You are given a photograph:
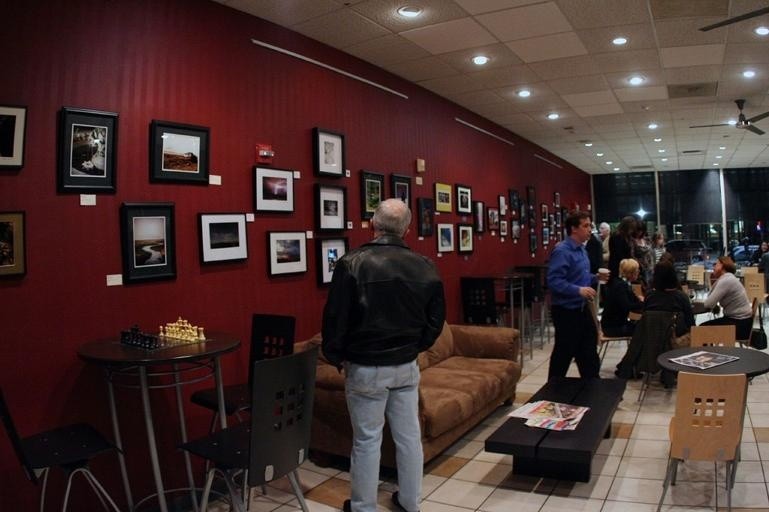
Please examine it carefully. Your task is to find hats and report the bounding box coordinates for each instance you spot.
[654,264,681,289]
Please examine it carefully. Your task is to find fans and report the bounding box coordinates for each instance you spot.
[696,5,767,30]
[688,99,769,139]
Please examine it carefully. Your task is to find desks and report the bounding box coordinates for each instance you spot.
[70,329,244,512]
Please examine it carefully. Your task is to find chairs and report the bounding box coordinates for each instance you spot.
[175,343,320,512]
[187,311,301,487]
[0,386,120,511]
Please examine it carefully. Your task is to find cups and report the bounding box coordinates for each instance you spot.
[598,268,611,284]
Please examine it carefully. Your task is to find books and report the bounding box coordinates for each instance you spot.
[507,400,591,431]
[670,351,740,369]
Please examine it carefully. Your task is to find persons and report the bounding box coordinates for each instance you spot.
[699,257,752,339]
[585,213,769,294]
[460,195,468,207]
[462,231,469,249]
[645,263,692,384]
[602,259,644,379]
[322,198,446,512]
[546,209,611,382]
[686,354,717,367]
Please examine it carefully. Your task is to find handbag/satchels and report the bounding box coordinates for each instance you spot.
[749,303,767,349]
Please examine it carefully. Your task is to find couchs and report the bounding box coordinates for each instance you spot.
[293,309,525,474]
